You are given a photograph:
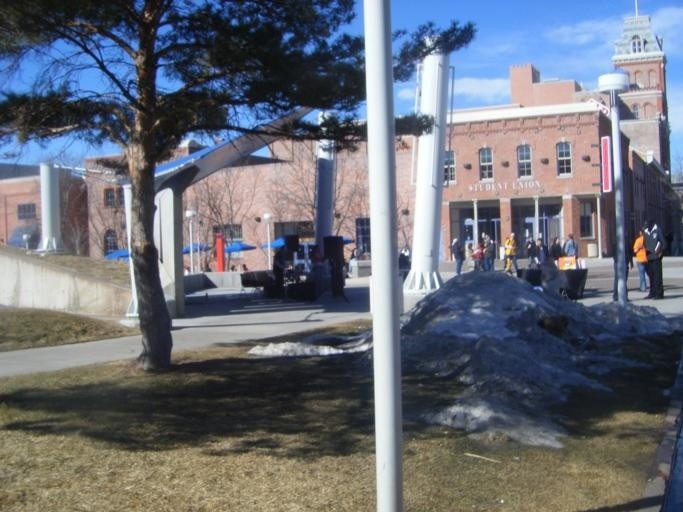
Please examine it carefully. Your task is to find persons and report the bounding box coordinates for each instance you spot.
[241,264,249,272]
[272,244,290,294]
[230,264,237,271]
[612,228,633,303]
[183,265,190,276]
[641,217,668,299]
[206,265,211,272]
[451,230,578,281]
[632,228,650,293]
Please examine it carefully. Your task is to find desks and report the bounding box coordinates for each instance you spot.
[517,267,587,297]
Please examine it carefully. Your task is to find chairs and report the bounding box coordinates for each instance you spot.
[239,270,275,301]
[556,271,569,298]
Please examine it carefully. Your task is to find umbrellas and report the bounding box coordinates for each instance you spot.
[183,242,209,254]
[305,236,355,249]
[224,239,258,253]
[106,249,131,261]
[261,238,306,253]
